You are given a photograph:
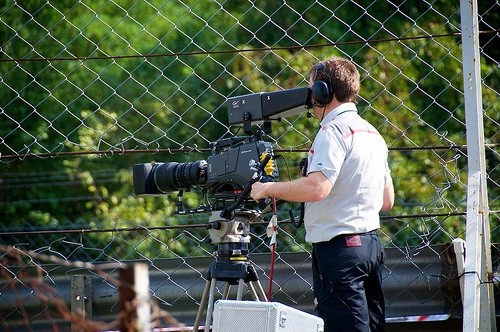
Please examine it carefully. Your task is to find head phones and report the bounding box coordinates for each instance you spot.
[312,59,333,106]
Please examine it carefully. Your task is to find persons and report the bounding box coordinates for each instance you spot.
[249,58,394,332]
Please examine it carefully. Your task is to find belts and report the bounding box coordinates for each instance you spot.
[355,228,380,235]
